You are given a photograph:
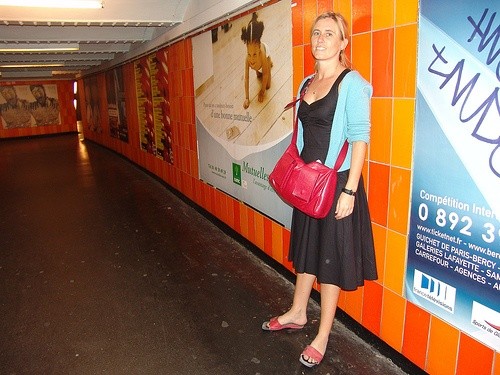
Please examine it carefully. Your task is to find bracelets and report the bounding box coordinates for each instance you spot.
[342,187,357,196]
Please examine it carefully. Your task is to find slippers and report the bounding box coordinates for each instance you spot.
[299,345,323,368]
[261,315,306,330]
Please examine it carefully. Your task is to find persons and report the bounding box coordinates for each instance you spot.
[262,11,378,368]
[240,12,273,109]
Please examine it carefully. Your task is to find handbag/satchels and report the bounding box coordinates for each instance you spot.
[268,143,338,219]
